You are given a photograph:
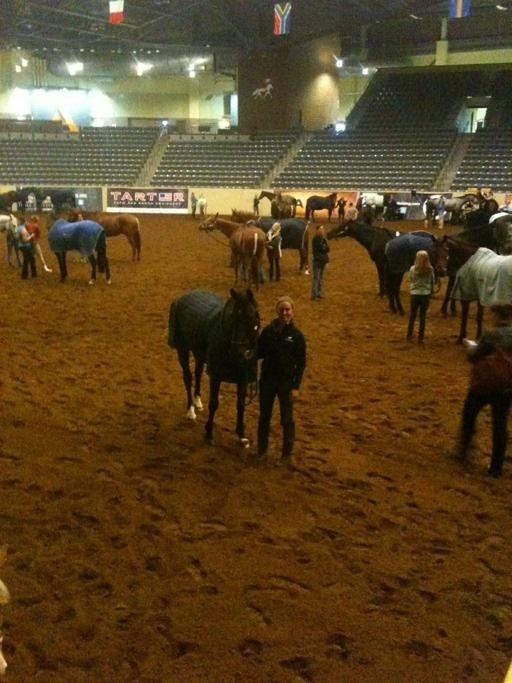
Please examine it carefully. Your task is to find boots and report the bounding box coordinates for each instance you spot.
[276,421,295,464]
[254,422,269,459]
[43,263,53,273]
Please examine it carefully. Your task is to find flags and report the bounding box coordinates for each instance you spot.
[273,3,293,35]
[108,0,127,24]
[448,0,471,17]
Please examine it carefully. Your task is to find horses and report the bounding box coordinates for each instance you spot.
[194,209,310,290]
[326,218,511,342]
[45,212,112,285]
[66,208,141,262]
[258,190,512,229]
[167,287,261,449]
[0,185,76,267]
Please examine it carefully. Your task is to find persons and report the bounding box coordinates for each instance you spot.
[266,221,282,282]
[25,214,54,272]
[18,218,36,279]
[255,294,306,464]
[189,191,197,218]
[406,250,435,343]
[197,193,207,215]
[311,224,329,300]
[335,196,347,223]
[346,192,512,228]
[451,304,511,477]
[254,194,260,215]
[8,212,22,268]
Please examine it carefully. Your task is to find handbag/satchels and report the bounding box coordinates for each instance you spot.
[18,240,31,248]
